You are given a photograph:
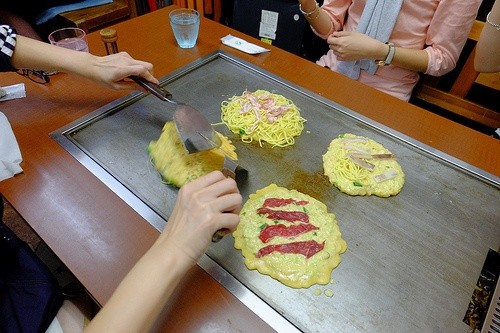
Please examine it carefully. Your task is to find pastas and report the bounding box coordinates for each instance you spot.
[335,155,370,183]
[210,88,308,148]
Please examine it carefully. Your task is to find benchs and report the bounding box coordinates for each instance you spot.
[408,18,499,137]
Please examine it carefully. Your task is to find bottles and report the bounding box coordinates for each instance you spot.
[100,28,121,54]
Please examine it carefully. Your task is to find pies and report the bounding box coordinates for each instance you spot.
[147,121,239,188]
[232,183,347,289]
[321,132,404,198]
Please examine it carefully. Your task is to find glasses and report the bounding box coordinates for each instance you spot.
[14,67,48,83]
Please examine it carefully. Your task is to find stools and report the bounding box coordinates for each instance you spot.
[57,2,130,34]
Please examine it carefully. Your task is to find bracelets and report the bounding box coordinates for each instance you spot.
[299,0,317,15]
[306,8,320,22]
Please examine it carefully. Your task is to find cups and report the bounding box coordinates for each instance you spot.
[47,28,90,54]
[168,8,200,48]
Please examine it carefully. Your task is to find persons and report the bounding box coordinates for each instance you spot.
[0,24,242,333]
[0,9,42,41]
[298,0,483,102]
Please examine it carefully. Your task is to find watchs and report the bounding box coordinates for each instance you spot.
[375,41,395,66]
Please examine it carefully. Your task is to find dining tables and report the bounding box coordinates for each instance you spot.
[0,0,500,333]
